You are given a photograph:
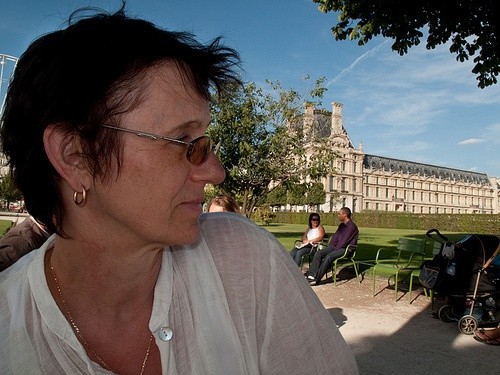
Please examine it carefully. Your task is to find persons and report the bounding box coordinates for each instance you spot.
[0,0,362,375]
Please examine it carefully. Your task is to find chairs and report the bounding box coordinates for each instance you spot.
[372,237,426,302]
[293,238,360,284]
[408,241,451,303]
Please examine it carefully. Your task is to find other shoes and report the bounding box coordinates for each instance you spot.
[304,271,319,286]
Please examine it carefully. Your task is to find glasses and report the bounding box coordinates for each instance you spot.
[312,218,319,221]
[99,122,222,166]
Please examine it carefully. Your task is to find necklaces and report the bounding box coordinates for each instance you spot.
[48,263,152,374]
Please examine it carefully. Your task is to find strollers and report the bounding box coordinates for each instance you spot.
[418,228,500,335]
